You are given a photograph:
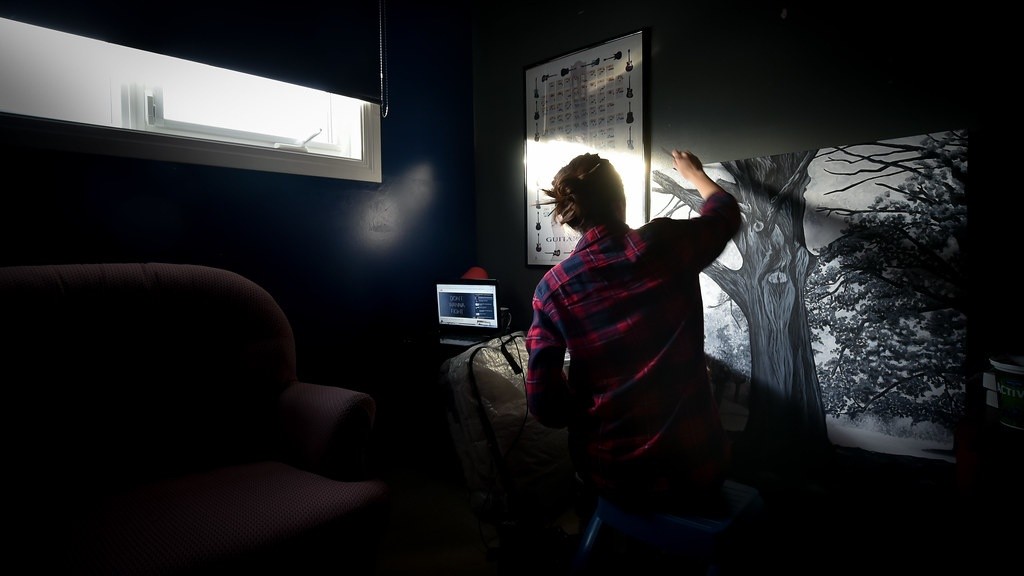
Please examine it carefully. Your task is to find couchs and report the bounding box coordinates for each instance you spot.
[1,263,390,575]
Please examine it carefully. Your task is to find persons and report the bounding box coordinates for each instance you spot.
[525,149,742,506]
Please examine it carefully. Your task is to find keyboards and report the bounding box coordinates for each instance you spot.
[439,339,482,346]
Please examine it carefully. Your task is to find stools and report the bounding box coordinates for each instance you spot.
[573,496,734,576]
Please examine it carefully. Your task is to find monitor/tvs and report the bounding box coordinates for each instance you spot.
[432,278,502,341]
